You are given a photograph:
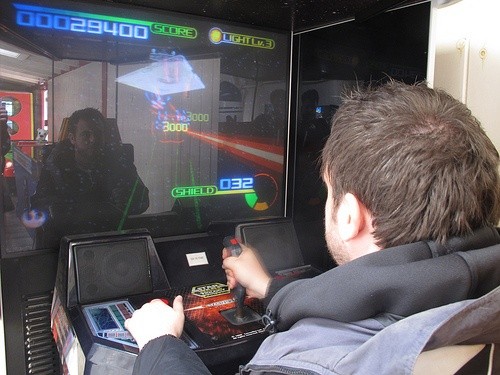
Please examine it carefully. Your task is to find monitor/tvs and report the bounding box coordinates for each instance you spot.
[0,0,293,260]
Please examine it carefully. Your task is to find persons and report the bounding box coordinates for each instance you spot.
[31,106,151,254]
[296,89,332,153]
[123,80,500,375]
[243,88,288,144]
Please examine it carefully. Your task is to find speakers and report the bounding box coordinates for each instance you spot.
[242,222,304,274]
[73,239,153,304]
